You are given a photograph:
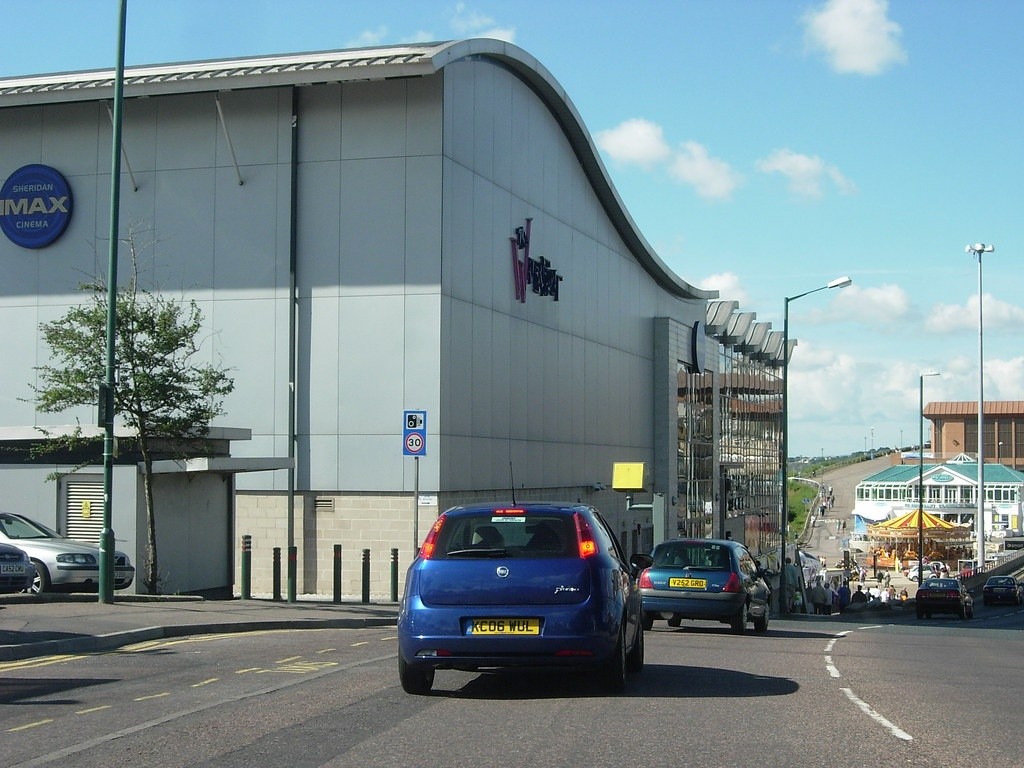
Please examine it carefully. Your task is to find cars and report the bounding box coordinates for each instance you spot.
[395,503,645,696]
[847,547,864,559]
[916,576,974,620]
[821,448,824,461]
[870,427,875,460]
[637,536,771,635]
[901,430,903,448]
[0,511,135,601]
[983,576,1020,606]
[907,560,949,582]
[864,436,867,452]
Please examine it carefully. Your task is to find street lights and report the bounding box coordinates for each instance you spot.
[780,272,852,614]
[917,369,942,589]
[964,239,996,570]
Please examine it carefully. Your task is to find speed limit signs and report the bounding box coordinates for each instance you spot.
[405,432,424,453]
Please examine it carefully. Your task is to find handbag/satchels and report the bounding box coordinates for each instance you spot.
[794,591,802,605]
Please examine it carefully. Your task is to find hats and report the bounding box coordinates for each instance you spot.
[824,583,830,587]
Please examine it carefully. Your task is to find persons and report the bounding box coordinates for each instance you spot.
[852,584,867,604]
[816,554,860,581]
[861,566,909,603]
[791,587,802,613]
[784,557,799,612]
[821,583,833,615]
[817,484,847,532]
[837,580,851,615]
[810,580,828,615]
[866,538,974,577]
